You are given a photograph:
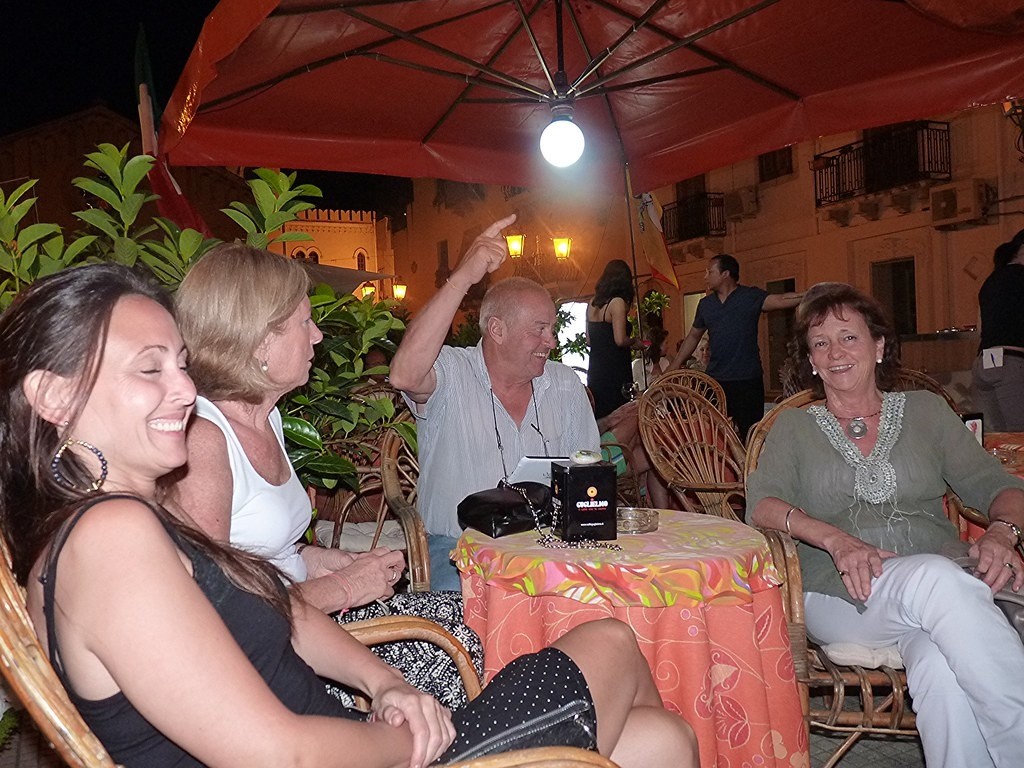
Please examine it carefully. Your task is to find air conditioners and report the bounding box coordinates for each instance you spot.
[928,179,986,228]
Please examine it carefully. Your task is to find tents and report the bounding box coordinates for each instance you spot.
[157,0,1024,199]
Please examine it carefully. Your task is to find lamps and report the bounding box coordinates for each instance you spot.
[724,184,757,220]
[1003,97,1023,118]
[361,281,377,299]
[504,221,528,259]
[552,225,574,261]
[392,275,408,302]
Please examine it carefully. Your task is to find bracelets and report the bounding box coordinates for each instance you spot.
[327,573,352,615]
[786,506,807,539]
[297,545,307,555]
[446,279,467,295]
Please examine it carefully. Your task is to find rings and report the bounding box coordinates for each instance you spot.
[839,571,849,576]
[1004,563,1013,569]
[393,570,397,580]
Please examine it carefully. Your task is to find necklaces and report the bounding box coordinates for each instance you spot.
[490,380,549,479]
[825,399,881,439]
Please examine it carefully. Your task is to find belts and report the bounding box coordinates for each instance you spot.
[977,349,1024,358]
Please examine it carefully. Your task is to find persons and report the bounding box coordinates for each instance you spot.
[172,244,485,713]
[689,332,711,371]
[596,401,669,508]
[585,259,646,418]
[968,228,1024,432]
[0,263,701,768]
[388,214,601,592]
[676,339,697,366]
[667,254,804,439]
[745,282,1024,768]
[631,327,669,391]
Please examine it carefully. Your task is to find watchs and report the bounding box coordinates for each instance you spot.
[992,519,1024,545]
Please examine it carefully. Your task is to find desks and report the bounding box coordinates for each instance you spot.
[942,433,1024,567]
[449,501,816,766]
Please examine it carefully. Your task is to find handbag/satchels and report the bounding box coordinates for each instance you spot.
[458,481,552,538]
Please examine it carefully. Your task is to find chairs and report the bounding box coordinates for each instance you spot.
[0,361,960,767]
